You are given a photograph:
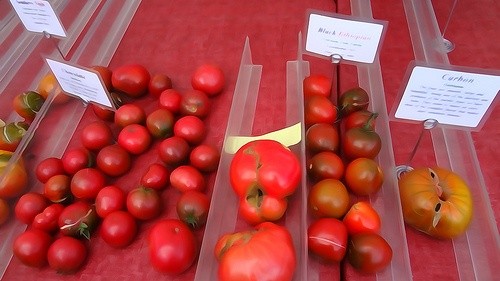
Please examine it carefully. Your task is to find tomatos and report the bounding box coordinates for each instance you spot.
[1,62,471,281]
